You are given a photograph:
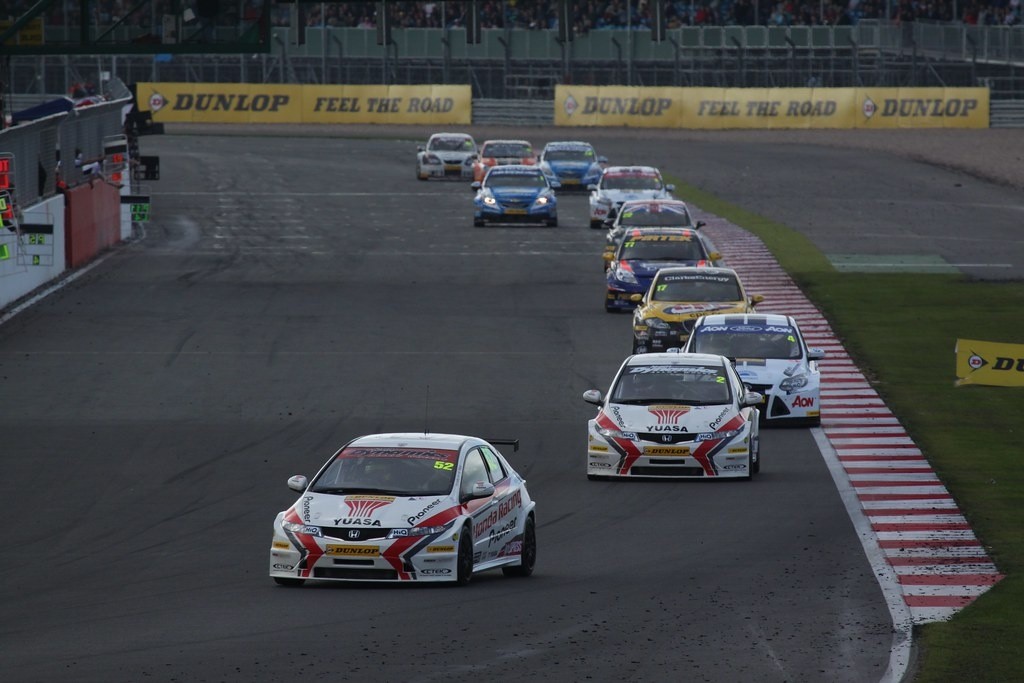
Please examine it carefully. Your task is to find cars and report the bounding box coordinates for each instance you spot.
[586,166,676,229]
[666,313,826,427]
[469,163,561,226]
[536,142,609,190]
[630,269,765,352]
[604,199,709,247]
[579,352,764,479]
[415,133,479,178]
[601,226,723,313]
[270,431,537,587]
[470,139,539,181]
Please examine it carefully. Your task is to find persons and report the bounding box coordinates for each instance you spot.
[703,332,736,360]
[0,0,1023,45]
[630,372,659,402]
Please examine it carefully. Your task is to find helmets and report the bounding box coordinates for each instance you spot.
[633,374,656,394]
[709,333,730,353]
